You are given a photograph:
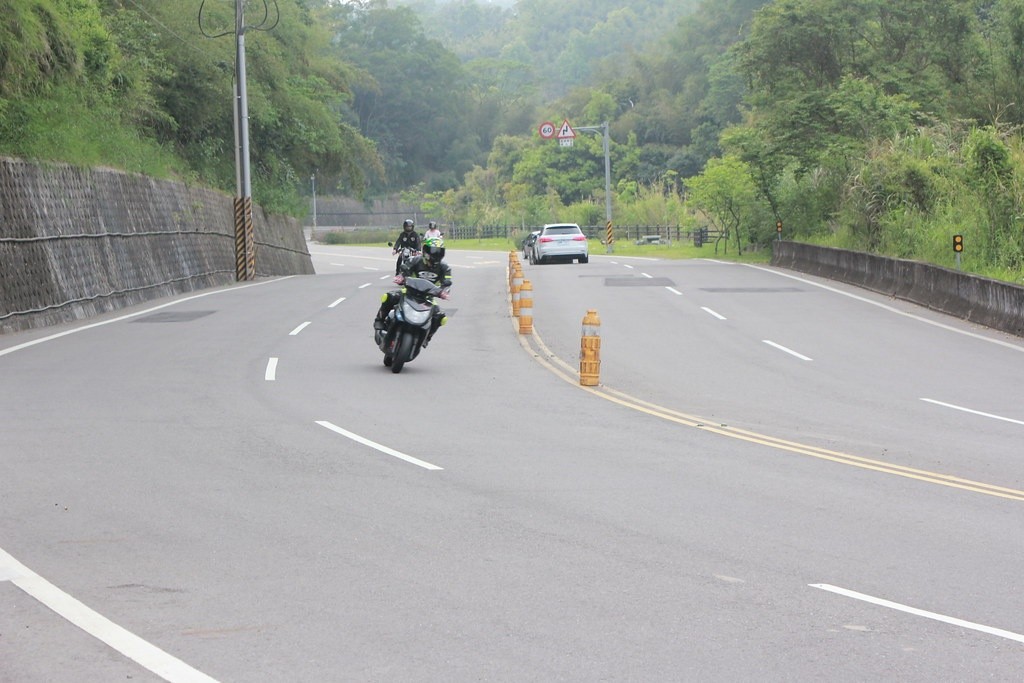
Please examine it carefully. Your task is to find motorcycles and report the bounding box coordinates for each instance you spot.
[388,233,445,265]
[373,265,452,373]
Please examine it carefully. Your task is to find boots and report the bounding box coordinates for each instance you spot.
[373,310,388,330]
[422,336,430,349]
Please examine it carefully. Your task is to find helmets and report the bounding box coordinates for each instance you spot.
[429,220,437,230]
[421,237,445,268]
[403,219,415,232]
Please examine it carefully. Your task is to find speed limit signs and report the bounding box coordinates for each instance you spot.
[540,123,556,139]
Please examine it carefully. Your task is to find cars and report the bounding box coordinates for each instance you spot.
[522,223,589,264]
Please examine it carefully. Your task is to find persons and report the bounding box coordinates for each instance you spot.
[422,221,440,240]
[392,219,421,276]
[374,237,451,348]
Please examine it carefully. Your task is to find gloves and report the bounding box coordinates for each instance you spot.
[392,250,396,255]
[440,292,447,300]
[394,275,404,284]
